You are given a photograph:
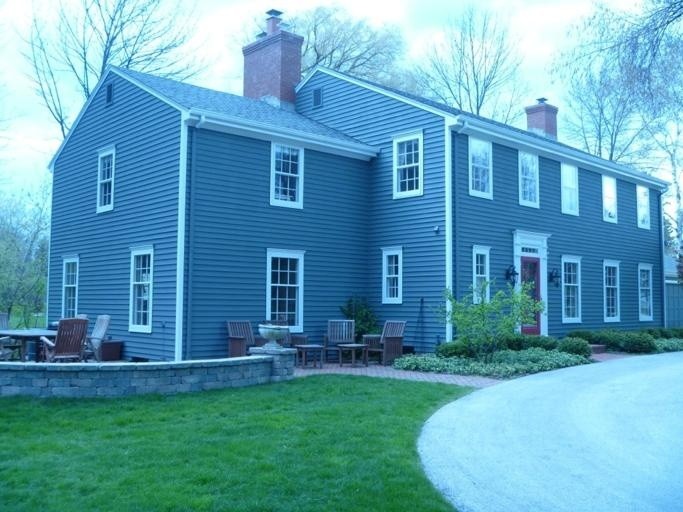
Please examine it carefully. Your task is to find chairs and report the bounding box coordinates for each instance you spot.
[225,320,308,365]
[0,313,111,363]
[323,320,407,367]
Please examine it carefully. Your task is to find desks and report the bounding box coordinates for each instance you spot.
[294,345,325,369]
